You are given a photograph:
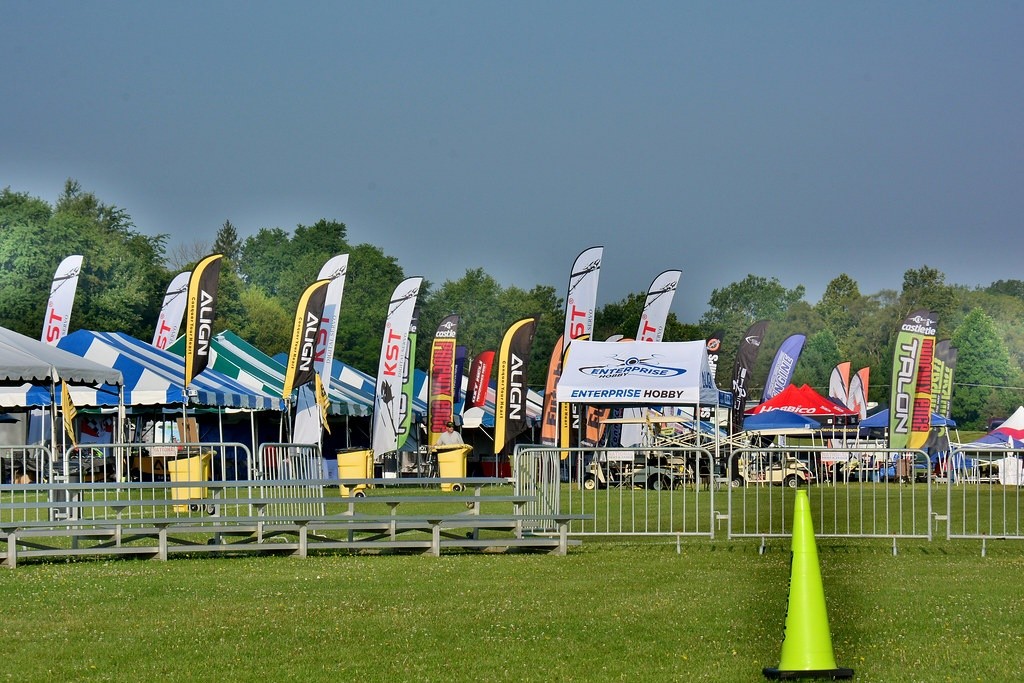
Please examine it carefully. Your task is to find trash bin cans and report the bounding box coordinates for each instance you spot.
[335,446,375,497]
[432,444,473,492]
[508,452,538,488]
[167,447,217,513]
[43,460,92,522]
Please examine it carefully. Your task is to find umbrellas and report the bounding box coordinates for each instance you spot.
[866,401,878,411]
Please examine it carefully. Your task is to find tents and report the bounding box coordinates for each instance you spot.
[742,383,859,486]
[555,339,733,487]
[953,405,1024,487]
[1,327,545,497]
[846,407,971,486]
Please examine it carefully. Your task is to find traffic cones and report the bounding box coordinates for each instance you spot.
[763,488,857,680]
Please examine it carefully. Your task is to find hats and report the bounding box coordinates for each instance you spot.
[447,422,454,426]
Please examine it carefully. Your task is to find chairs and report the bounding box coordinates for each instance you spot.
[609,454,720,492]
